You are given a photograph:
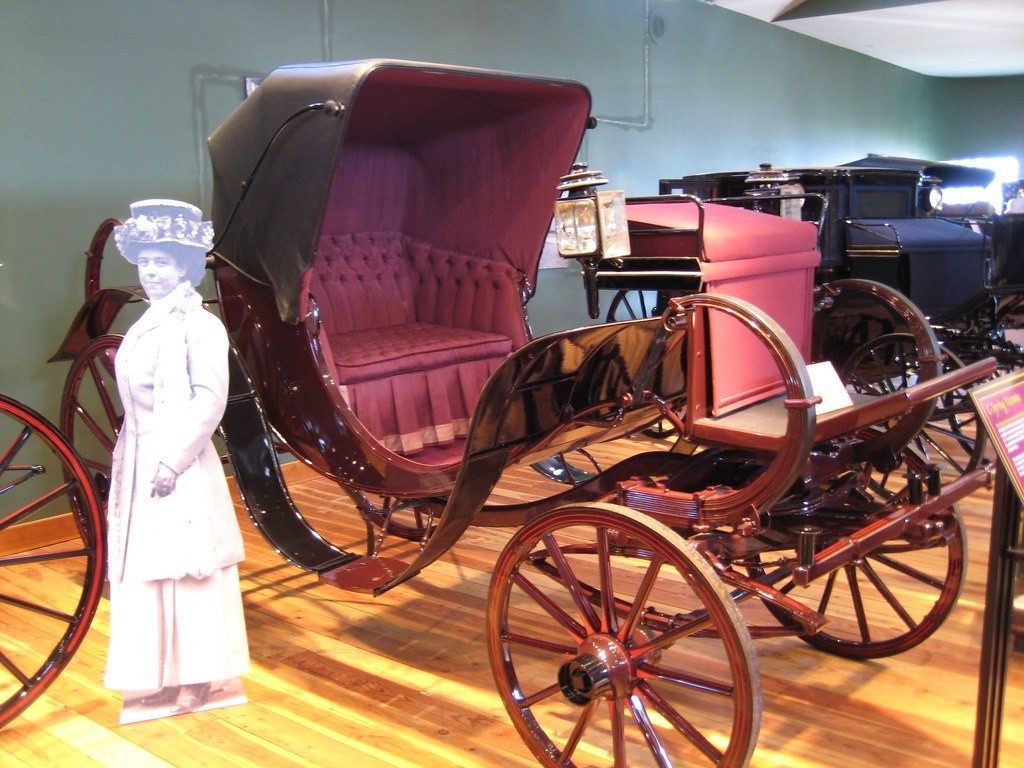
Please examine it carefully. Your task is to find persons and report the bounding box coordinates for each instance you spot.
[107,199,251,725]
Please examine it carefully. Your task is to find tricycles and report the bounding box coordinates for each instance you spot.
[30,59,1003,768]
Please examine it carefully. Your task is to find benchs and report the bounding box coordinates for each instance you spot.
[312,231,526,454]
[846,218,993,323]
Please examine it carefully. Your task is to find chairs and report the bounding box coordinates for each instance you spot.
[626,189,829,420]
